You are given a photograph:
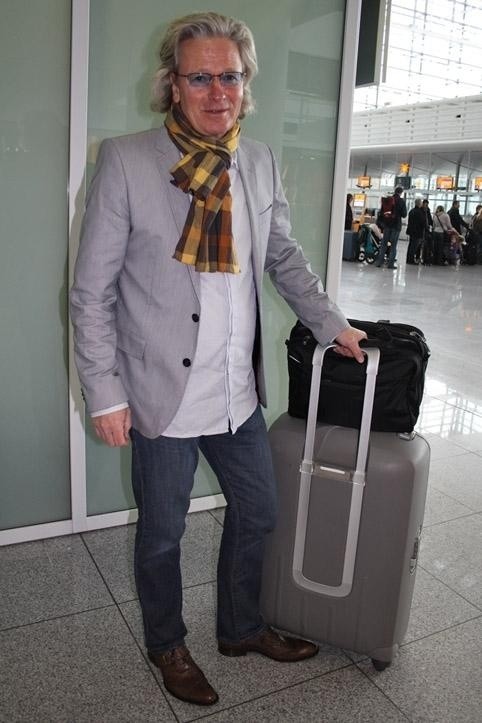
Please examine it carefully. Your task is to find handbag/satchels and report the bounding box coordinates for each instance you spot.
[287,320,430,434]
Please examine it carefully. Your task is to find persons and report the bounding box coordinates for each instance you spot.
[405,199,482,266]
[375,187,407,269]
[68,11,367,706]
[345,193,357,230]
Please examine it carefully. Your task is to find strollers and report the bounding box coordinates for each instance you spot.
[358,222,392,265]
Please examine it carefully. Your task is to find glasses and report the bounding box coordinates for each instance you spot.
[173,71,248,87]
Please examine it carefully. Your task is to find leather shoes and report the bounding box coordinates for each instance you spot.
[149,641,219,706]
[219,627,319,662]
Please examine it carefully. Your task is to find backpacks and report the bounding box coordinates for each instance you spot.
[381,196,396,223]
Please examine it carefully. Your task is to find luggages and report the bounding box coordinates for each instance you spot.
[343,230,360,262]
[261,342,432,671]
